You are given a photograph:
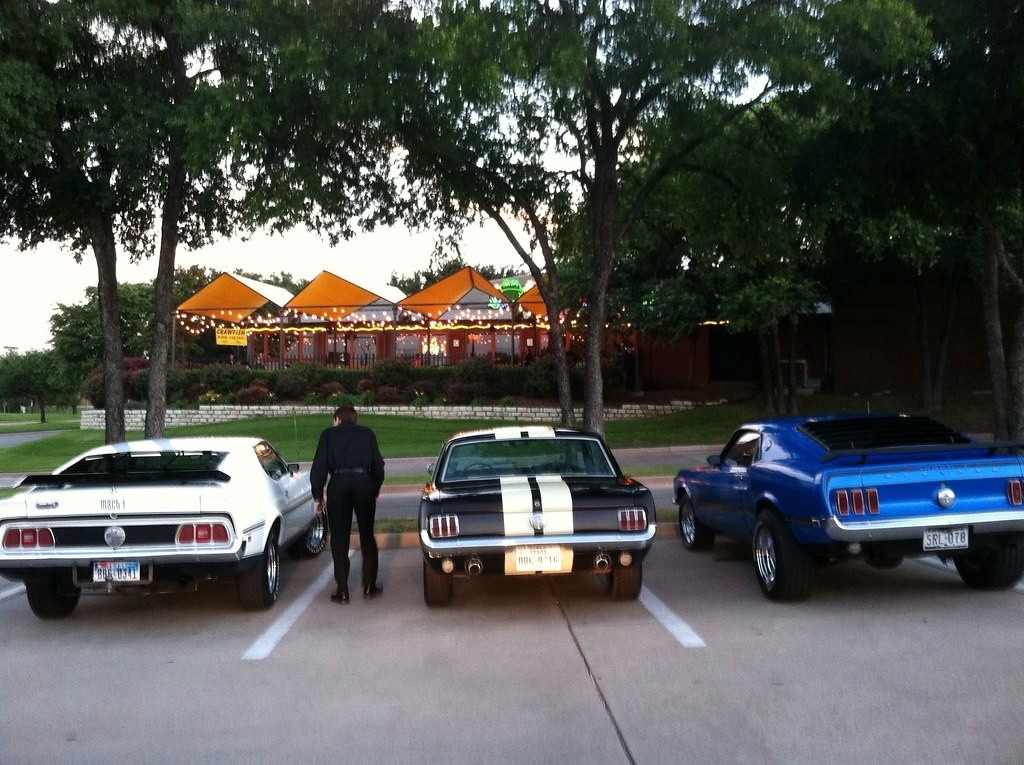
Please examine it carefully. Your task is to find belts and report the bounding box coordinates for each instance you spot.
[334,467,366,473]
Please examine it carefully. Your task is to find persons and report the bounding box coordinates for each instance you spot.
[310,407,385,604]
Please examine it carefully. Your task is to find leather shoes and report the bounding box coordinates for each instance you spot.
[332,590,350,604]
[364,585,383,600]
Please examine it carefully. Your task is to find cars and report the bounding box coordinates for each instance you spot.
[0,436,330,620]
[673,413,1024,603]
[417,426,657,609]
